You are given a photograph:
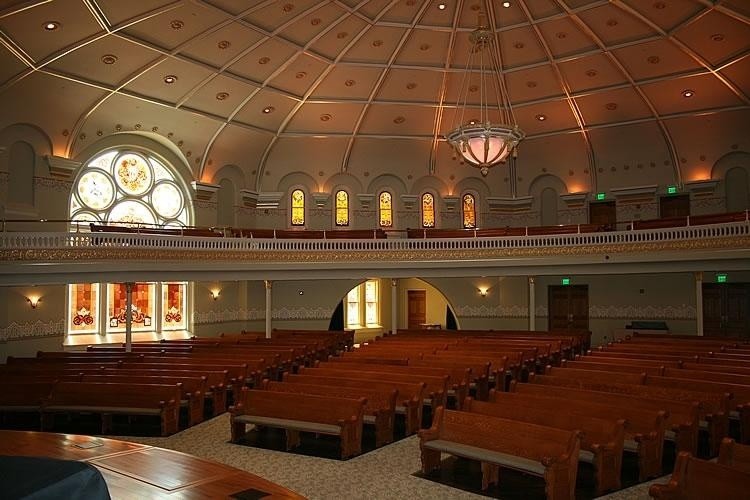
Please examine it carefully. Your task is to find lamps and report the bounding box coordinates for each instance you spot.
[445,1,524,178]
